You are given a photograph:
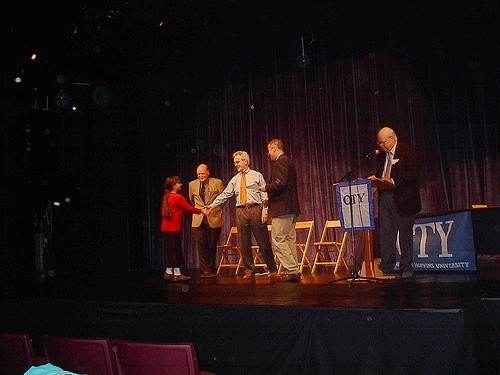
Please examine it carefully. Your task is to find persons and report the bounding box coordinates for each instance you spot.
[161,175,208,276]
[259,139,301,282]
[369,127,422,277]
[189,164,225,278]
[203,151,278,278]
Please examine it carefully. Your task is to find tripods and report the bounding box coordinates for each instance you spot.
[328,153,386,286]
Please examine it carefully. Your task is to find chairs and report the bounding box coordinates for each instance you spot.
[310,219,349,274]
[251,225,273,276]
[0,334,213,375]
[277,221,313,274]
[216,226,242,276]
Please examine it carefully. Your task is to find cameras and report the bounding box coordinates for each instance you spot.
[365,149,380,158]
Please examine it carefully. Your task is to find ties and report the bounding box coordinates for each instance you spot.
[240,173,246,205]
[384,152,392,178]
[200,183,206,204]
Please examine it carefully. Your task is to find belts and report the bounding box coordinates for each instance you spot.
[239,203,259,208]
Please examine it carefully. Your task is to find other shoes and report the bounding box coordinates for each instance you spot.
[162,272,174,280]
[402,270,413,278]
[267,271,277,277]
[243,274,254,279]
[278,272,301,281]
[173,274,191,281]
[201,272,217,278]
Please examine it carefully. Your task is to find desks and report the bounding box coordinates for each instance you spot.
[392,206,500,270]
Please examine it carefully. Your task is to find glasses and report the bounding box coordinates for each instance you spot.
[377,136,392,145]
